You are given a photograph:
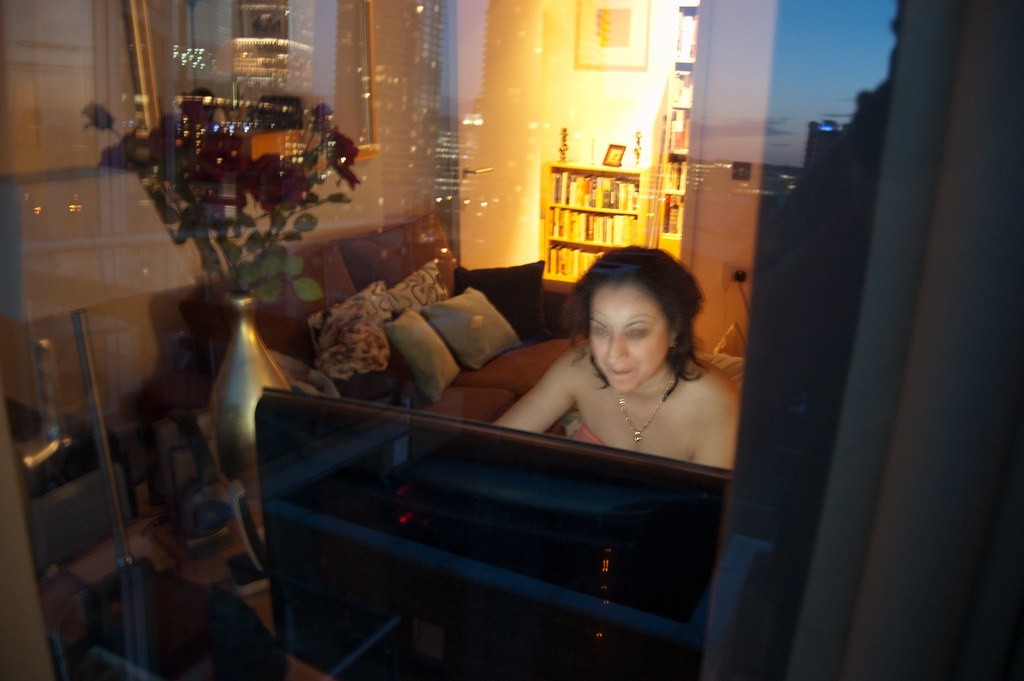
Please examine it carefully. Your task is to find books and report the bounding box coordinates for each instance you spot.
[663,15,694,234]
[549,174,639,278]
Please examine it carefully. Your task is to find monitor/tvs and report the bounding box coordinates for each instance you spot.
[252,387,732,681]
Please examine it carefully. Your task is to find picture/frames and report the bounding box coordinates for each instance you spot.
[602,144,626,167]
[119,0,381,164]
[573,0,651,72]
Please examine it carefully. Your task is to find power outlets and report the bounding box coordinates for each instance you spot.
[720,263,752,289]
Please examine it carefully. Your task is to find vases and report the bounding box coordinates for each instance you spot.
[204,295,292,500]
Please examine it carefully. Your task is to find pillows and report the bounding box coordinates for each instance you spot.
[421,286,522,371]
[307,280,391,381]
[453,259,551,341]
[383,307,461,406]
[385,258,449,314]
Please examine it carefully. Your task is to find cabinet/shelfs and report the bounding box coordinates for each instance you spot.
[656,4,698,258]
[540,163,649,284]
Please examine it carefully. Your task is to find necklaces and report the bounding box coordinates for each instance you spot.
[618,372,674,442]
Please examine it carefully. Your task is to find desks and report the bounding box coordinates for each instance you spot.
[40,472,334,681]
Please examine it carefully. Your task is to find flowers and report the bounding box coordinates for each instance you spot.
[80,86,361,301]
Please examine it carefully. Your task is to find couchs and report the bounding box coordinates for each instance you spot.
[177,214,590,435]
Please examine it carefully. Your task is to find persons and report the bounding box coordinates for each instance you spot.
[490,246,740,470]
